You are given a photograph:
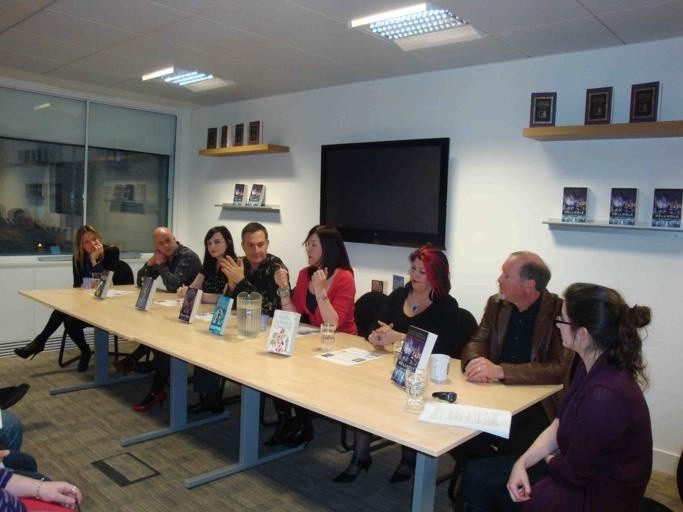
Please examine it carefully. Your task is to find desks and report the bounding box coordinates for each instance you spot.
[17,285,565,511]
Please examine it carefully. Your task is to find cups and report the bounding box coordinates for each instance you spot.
[430,353,451,382]
[392,341,404,365]
[92,272,101,290]
[83,277,93,291]
[319,323,337,350]
[261,314,269,331]
[235,291,263,338]
[403,367,426,412]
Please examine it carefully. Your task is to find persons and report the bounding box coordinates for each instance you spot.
[0,382,84,511]
[117,226,200,374]
[329,244,458,485]
[186,222,290,415]
[14,225,120,372]
[262,223,359,448]
[131,223,236,411]
[445,251,575,467]
[453,282,652,512]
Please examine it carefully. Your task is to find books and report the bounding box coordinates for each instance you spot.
[264,309,301,356]
[248,121,262,145]
[233,123,245,147]
[560,187,590,224]
[390,324,438,390]
[134,277,157,310]
[220,125,230,147]
[207,127,218,149]
[93,269,114,298]
[649,187,682,229]
[245,184,266,207]
[608,186,639,226]
[177,286,202,323]
[232,183,247,206]
[206,294,233,336]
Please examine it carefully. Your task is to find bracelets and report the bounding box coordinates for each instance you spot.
[276,285,290,297]
[280,301,290,306]
[316,294,327,304]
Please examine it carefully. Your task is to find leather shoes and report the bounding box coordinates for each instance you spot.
[277,411,292,436]
[388,458,416,485]
[136,360,157,372]
[187,394,221,415]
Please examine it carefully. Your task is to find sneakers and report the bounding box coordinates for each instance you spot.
[0,382,31,410]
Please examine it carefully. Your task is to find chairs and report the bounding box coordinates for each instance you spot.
[641,452,682,511]
[369,308,478,486]
[58,260,134,368]
[260,290,388,451]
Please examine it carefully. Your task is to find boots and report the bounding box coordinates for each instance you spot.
[15,334,47,360]
[283,413,316,451]
[75,344,92,372]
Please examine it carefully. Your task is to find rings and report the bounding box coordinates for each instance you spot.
[477,366,479,372]
[378,335,381,341]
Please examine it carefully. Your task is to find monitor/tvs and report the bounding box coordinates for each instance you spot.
[319,136,450,252]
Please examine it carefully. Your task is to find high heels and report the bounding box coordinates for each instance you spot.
[332,454,373,483]
[134,391,167,411]
[112,357,135,375]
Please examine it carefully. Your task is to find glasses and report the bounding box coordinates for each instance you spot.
[553,315,579,328]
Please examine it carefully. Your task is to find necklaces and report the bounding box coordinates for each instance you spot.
[410,292,428,312]
